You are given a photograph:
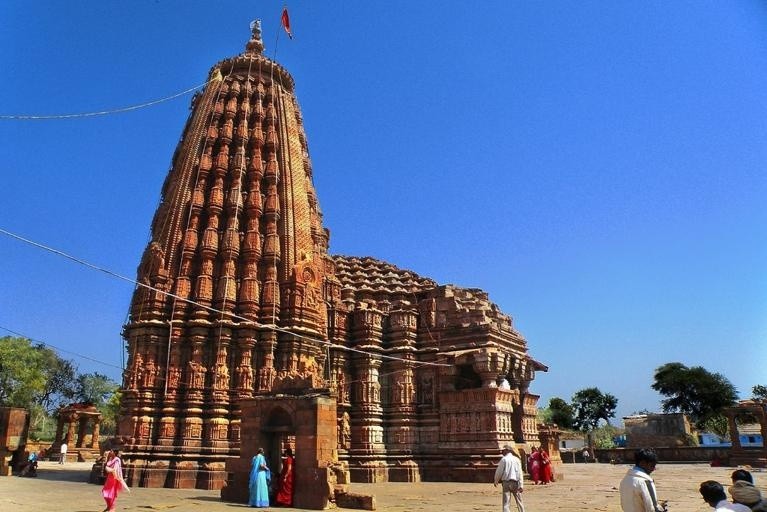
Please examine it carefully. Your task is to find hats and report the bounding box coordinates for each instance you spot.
[729,481,761,504]
[500,445,511,450]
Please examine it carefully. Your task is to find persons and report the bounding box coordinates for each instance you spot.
[620,448,669,512]
[28,449,48,463]
[582,449,590,463]
[538,446,552,485]
[493,444,525,512]
[102,448,131,512]
[528,446,540,486]
[274,448,295,507]
[249,447,274,508]
[58,441,67,464]
[699,470,767,512]
